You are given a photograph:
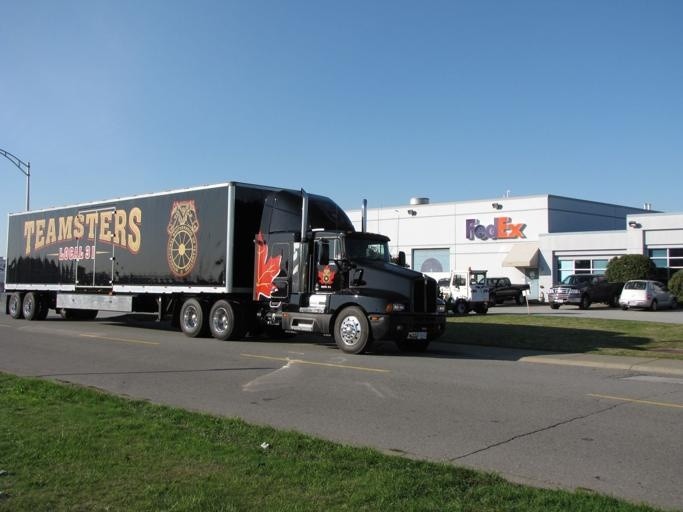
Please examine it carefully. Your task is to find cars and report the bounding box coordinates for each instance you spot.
[618,279,681,312]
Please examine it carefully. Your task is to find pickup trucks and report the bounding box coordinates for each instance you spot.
[544,273,623,309]
[475,276,533,310]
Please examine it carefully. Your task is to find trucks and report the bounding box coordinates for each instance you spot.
[5,178,450,359]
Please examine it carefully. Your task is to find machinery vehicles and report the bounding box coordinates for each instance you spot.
[421,265,491,317]
